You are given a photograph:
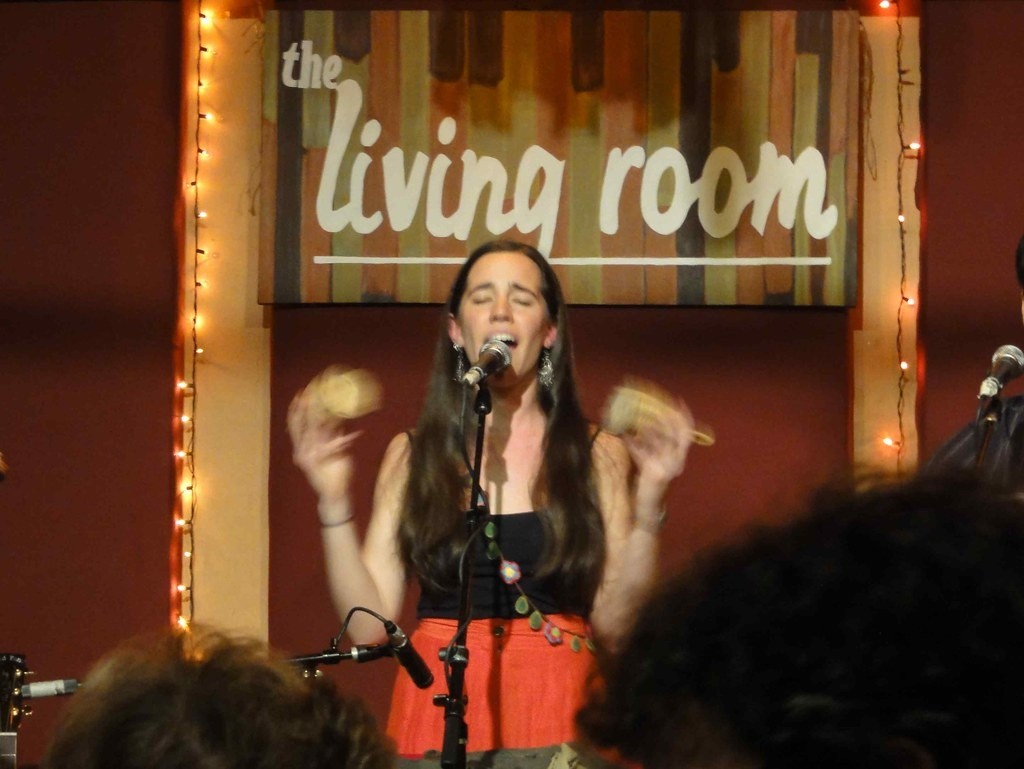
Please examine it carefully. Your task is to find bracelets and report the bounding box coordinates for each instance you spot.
[315,498,355,527]
[635,505,669,525]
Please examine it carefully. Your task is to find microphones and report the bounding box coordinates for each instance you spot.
[978,344,1024,401]
[463,339,513,387]
[384,621,434,689]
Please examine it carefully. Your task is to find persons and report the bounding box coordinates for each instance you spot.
[288,242,677,769]
[921,233,1024,495]
[579,467,1024,768]
[36,623,399,769]
